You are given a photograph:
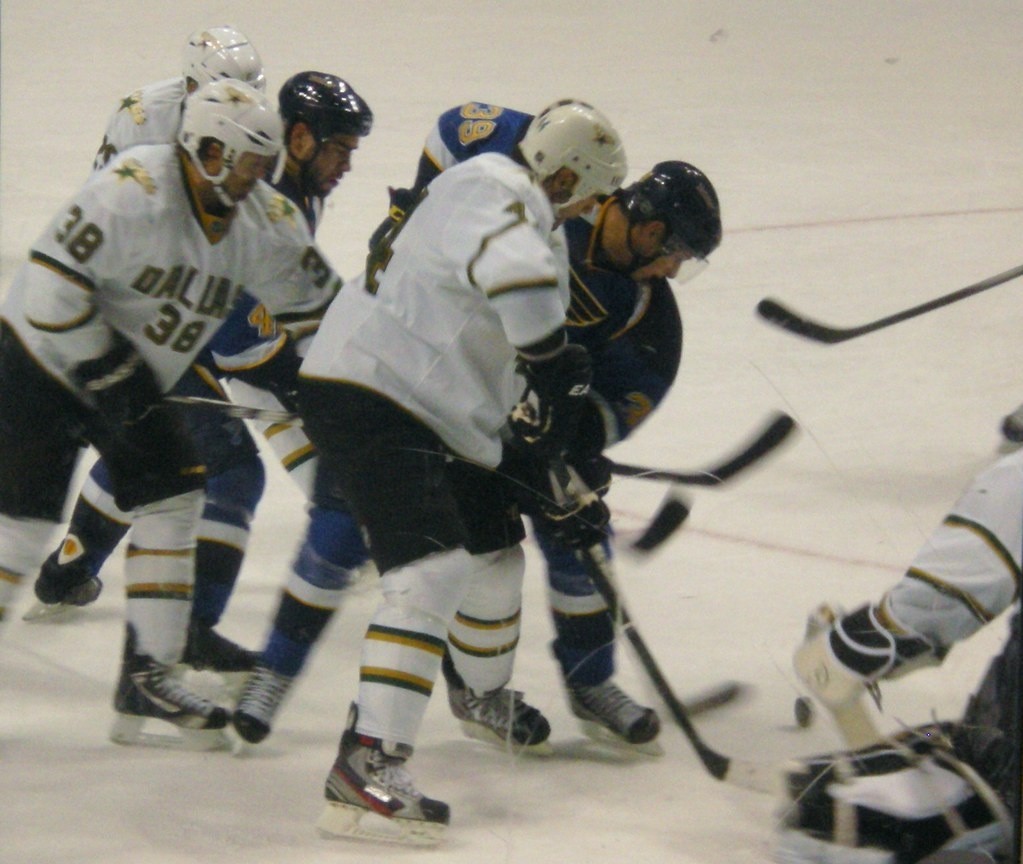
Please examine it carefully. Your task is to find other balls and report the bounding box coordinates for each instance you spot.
[795,696,815,729]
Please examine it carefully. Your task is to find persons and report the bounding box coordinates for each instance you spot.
[21,73,374,697]
[88,27,264,186]
[773,447,1023,864]
[225,104,724,759]
[299,100,628,848]
[0,75,345,752]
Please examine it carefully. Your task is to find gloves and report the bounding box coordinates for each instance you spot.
[514,328,613,463]
[370,184,422,249]
[242,349,304,412]
[526,455,610,543]
[72,329,163,425]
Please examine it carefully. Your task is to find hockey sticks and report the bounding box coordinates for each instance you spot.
[169,389,800,488]
[603,485,696,553]
[594,542,748,722]
[754,264,1023,347]
[575,533,794,795]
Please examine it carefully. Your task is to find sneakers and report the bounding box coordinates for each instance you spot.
[230,667,289,756]
[110,620,230,754]
[175,619,263,698]
[23,528,103,620]
[316,702,451,849]
[441,671,555,757]
[568,681,663,757]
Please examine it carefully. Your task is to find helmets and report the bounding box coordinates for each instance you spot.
[178,77,279,169]
[279,71,373,149]
[630,165,723,259]
[183,25,263,91]
[519,98,628,202]
